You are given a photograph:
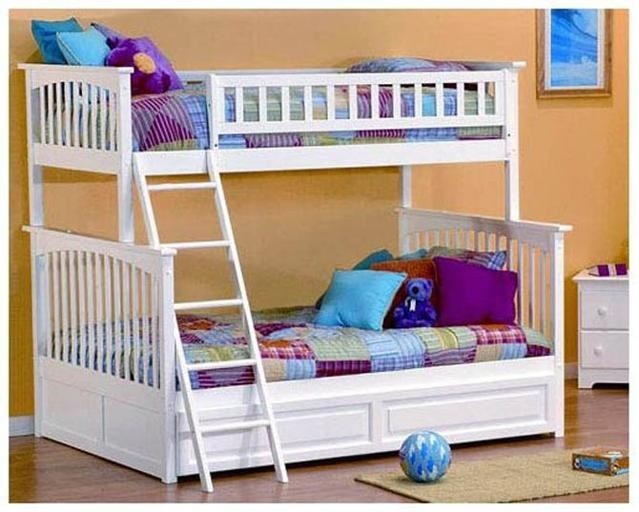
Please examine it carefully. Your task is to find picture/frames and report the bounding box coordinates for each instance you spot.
[534,9,614,98]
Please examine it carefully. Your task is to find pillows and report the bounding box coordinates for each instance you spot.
[309,244,522,334]
[29,15,183,96]
[345,55,475,72]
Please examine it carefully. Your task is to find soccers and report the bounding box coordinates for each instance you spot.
[401,430,451,481]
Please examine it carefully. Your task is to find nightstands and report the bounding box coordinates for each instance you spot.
[570,265,629,390]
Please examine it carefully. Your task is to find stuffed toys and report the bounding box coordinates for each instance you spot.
[393,277,438,328]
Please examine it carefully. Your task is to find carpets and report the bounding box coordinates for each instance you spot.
[351,444,629,501]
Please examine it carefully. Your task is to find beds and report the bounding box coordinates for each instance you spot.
[17,62,574,486]
